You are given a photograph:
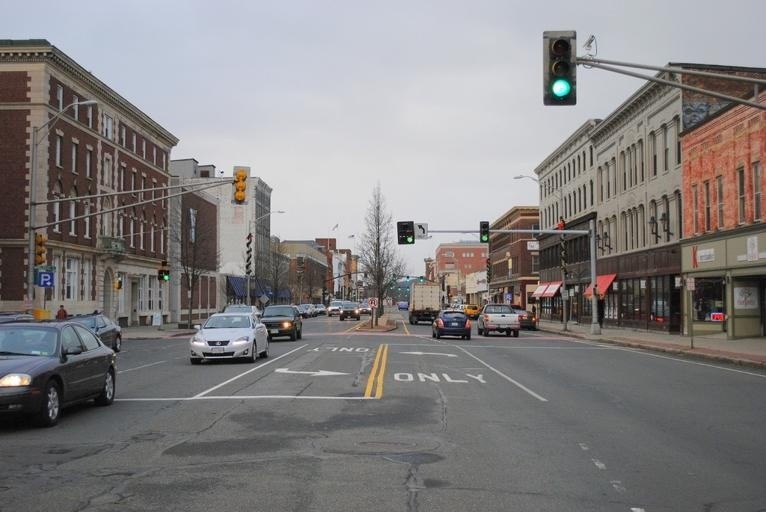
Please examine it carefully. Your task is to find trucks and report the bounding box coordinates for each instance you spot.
[408,282,444,325]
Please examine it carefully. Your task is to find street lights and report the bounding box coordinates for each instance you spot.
[26,99,99,311]
[246,211,287,306]
[513,174,569,220]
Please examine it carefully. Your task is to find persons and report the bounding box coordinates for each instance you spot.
[55,303,66,320]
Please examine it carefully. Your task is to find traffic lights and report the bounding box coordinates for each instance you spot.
[420,276,424,283]
[407,276,410,282]
[542,31,580,107]
[35,232,48,266]
[480,221,490,243]
[397,221,415,245]
[158,270,170,282]
[393,274,396,283]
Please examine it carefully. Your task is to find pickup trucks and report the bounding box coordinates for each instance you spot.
[477,303,521,337]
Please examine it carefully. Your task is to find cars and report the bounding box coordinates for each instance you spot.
[397,300,408,310]
[0,310,123,427]
[432,309,471,340]
[514,309,537,330]
[292,300,373,321]
[189,313,270,365]
[445,303,480,319]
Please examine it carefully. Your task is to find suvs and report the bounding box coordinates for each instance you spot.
[218,304,303,342]
